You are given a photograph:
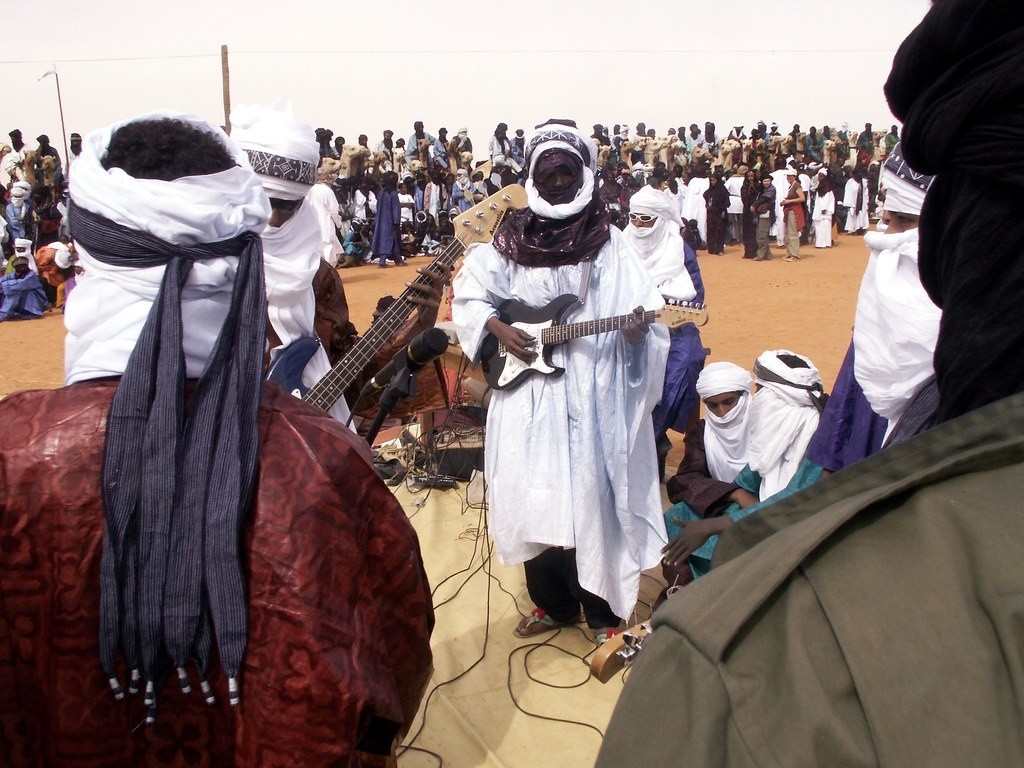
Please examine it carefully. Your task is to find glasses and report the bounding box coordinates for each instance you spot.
[627,211,657,222]
[271,199,302,214]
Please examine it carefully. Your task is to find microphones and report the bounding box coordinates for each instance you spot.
[361,327,448,397]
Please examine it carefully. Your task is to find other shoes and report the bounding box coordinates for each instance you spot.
[754,256,763,261]
[783,256,798,262]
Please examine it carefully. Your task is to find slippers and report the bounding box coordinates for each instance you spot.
[596,627,618,648]
[513,606,583,638]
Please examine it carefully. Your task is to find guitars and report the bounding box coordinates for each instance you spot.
[261,170,531,413]
[479,293,710,393]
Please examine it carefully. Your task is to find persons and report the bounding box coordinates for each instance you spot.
[316,121,527,267]
[0,257,49,322]
[648,350,831,619]
[0,127,84,270]
[589,0,1024,768]
[222,105,453,431]
[616,185,706,481]
[34,234,72,308]
[812,168,835,248]
[454,119,670,648]
[0,111,434,768]
[740,169,761,258]
[4,239,39,279]
[780,169,805,261]
[373,171,406,266]
[304,168,344,267]
[703,173,730,256]
[806,140,942,481]
[750,174,776,260]
[587,119,900,246]
